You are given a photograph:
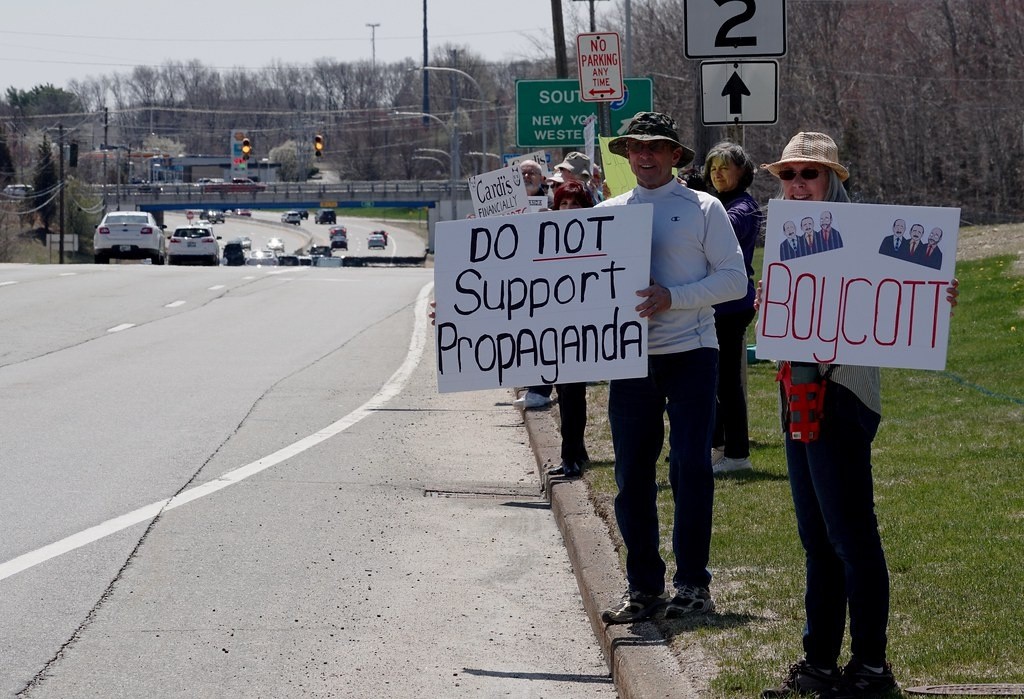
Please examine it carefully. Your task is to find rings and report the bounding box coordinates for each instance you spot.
[654,304,657,309]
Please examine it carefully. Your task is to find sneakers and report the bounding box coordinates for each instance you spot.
[602,589,655,623]
[813,659,902,699]
[758,658,843,699]
[665,584,715,617]
[513,392,552,407]
[712,457,753,472]
[711,447,724,465]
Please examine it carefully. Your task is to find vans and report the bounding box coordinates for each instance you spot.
[4,185,34,195]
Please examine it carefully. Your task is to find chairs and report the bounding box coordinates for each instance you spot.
[112,219,121,223]
[181,231,187,237]
[137,219,144,222]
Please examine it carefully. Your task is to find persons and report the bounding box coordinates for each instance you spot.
[429,112,748,623]
[702,141,762,474]
[467,152,610,408]
[548,181,596,478]
[753,132,960,699]
[665,167,707,462]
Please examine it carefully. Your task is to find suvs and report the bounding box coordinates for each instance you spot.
[314,209,337,224]
[281,208,309,226]
[232,178,254,183]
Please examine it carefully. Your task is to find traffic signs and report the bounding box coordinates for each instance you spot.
[576,33,624,103]
[516,78,652,150]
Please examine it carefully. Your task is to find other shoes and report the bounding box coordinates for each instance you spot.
[548,462,565,475]
[562,461,587,479]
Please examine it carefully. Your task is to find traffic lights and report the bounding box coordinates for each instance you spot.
[70,143,78,167]
[314,135,324,158]
[242,138,252,160]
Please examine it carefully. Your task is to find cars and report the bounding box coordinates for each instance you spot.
[200,209,226,224]
[197,178,223,183]
[222,236,368,267]
[329,226,347,241]
[168,226,223,266]
[94,211,168,265]
[234,209,251,216]
[138,185,163,192]
[367,230,388,249]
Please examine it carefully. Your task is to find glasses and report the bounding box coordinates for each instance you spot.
[541,184,549,190]
[550,183,562,189]
[777,168,825,181]
[628,140,666,154]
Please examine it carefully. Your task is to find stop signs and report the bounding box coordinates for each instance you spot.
[187,211,194,220]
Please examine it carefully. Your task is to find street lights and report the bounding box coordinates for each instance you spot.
[366,23,381,70]
[387,65,485,179]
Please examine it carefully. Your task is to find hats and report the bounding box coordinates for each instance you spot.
[760,132,850,182]
[607,112,696,168]
[554,152,593,178]
[547,171,564,182]
